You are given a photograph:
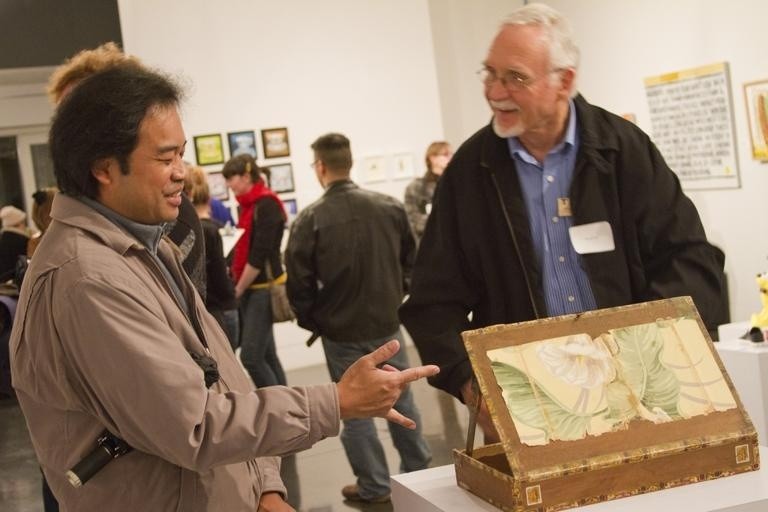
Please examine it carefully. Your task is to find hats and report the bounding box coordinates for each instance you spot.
[0,205,26,227]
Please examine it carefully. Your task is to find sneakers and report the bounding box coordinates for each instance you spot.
[342,485,390,503]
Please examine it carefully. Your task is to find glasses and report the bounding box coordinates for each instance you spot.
[476,65,546,88]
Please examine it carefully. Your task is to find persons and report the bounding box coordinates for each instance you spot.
[27,187,60,262]
[183,166,240,354]
[0,205,31,283]
[400,3,730,443]
[285,133,433,504]
[224,153,288,389]
[184,159,236,227]
[405,142,454,251]
[47,41,208,305]
[9,69,440,512]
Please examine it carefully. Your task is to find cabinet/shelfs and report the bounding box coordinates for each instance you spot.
[711,319,767,446]
[389,446,767,511]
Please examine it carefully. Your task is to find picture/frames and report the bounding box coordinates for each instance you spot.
[262,127,291,159]
[206,170,230,201]
[281,199,299,214]
[266,163,295,194]
[744,79,767,162]
[193,134,224,165]
[227,131,257,160]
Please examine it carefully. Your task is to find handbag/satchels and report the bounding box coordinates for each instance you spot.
[254,201,295,323]
